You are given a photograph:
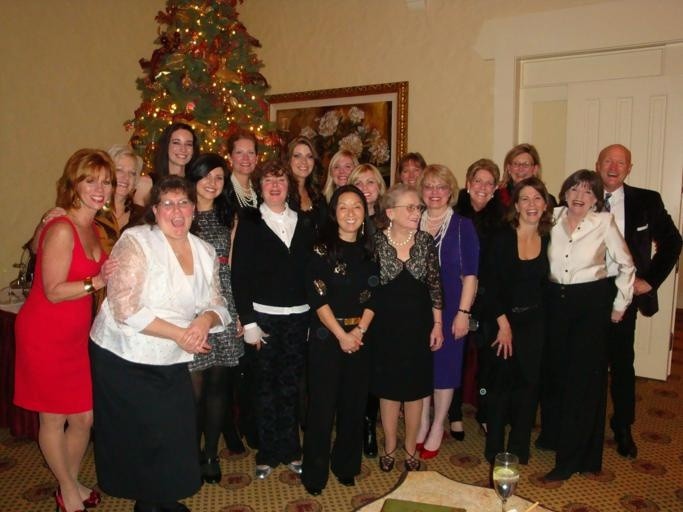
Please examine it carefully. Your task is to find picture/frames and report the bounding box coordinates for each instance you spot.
[269,80,408,193]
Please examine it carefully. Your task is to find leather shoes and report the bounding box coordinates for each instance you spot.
[225,432,246,456]
[256,462,270,479]
[449,419,465,442]
[202,451,222,484]
[364,427,378,459]
[611,415,637,458]
[333,466,358,486]
[299,474,325,496]
[417,427,446,458]
[286,460,304,474]
[473,410,605,478]
[55,484,100,511]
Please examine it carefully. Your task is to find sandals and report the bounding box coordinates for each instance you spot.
[379,445,396,471]
[402,445,421,472]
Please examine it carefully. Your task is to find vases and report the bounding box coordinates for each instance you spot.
[316,151,332,190]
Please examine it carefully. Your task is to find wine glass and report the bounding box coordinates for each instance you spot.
[493,452,519,512]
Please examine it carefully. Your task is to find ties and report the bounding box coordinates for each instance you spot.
[604,193,611,212]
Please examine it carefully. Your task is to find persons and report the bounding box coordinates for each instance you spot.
[109,142,144,228]
[593,143,683,461]
[544,169,636,478]
[88,175,232,511]
[15,151,122,512]
[130,120,554,491]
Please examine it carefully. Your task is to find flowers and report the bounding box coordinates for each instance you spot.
[296,105,390,167]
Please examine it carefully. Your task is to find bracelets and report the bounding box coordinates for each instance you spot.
[84,274,98,295]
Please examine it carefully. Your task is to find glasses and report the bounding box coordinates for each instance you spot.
[161,198,189,208]
[394,204,424,213]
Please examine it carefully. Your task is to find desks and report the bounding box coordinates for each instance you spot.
[0,285,40,441]
